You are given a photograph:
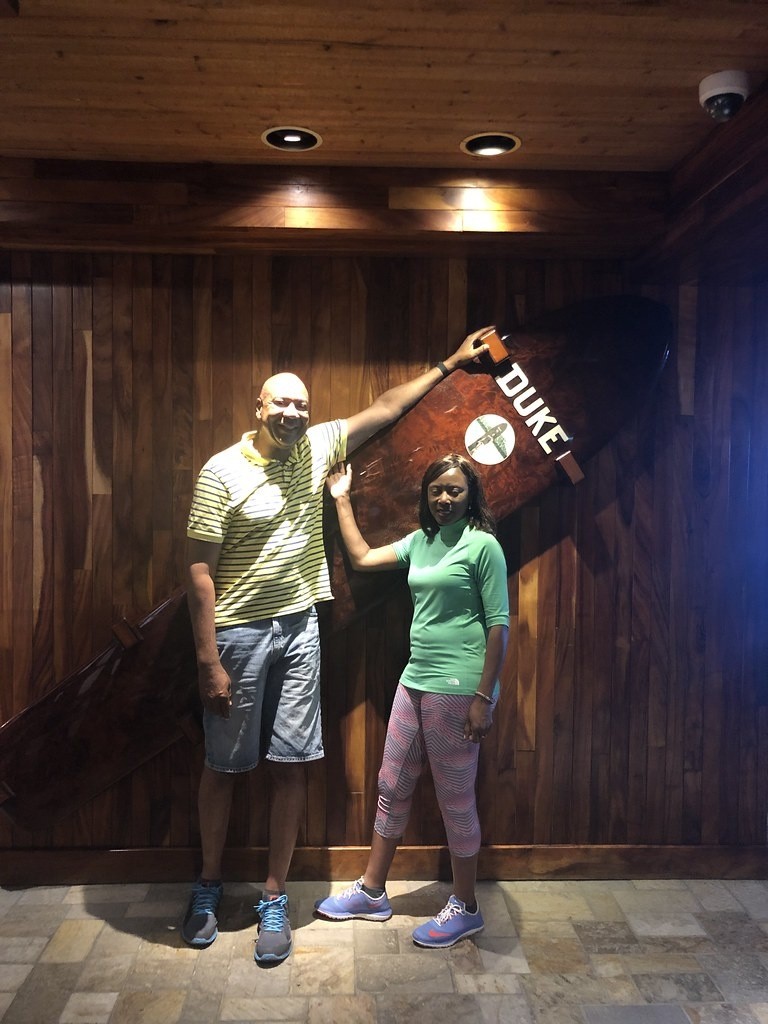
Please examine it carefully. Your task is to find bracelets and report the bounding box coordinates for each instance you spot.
[437,362,450,376]
[475,691,493,705]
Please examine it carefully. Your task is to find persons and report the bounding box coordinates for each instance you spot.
[182,325,495,961]
[315,453,511,947]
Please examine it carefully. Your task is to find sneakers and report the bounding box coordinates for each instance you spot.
[181,880,224,945]
[253,894,293,961]
[411,895,485,948]
[314,875,392,920]
